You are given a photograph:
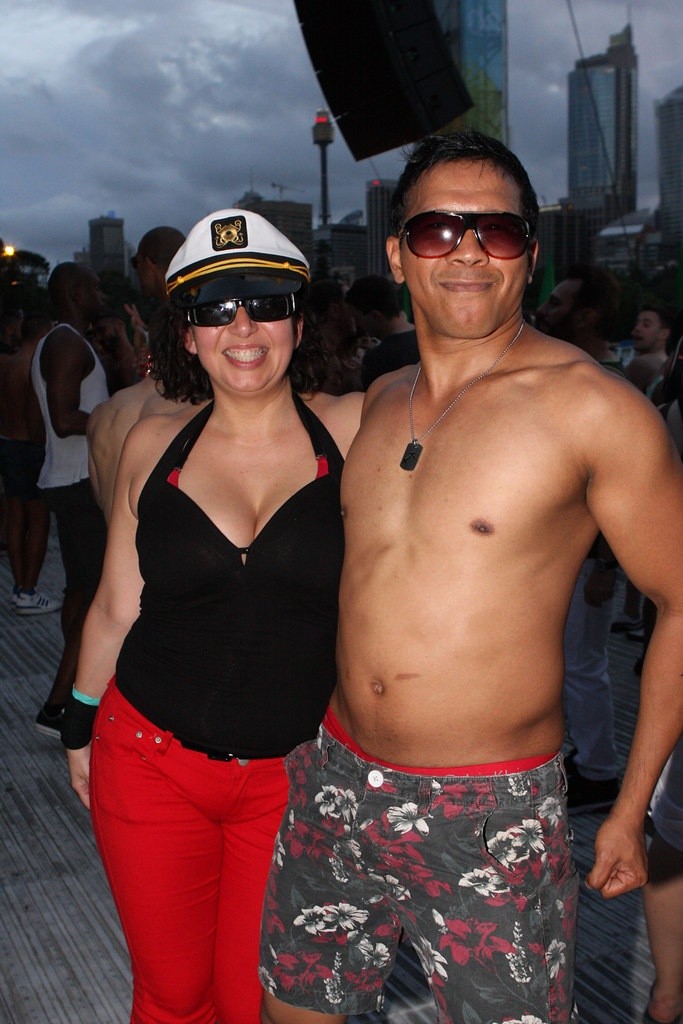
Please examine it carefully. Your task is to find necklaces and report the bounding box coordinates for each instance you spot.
[400,322,525,471]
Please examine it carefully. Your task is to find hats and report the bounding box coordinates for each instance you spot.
[164,208,310,308]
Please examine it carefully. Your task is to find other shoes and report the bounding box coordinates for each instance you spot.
[559,757,621,815]
[33,703,64,740]
[626,626,645,640]
[611,611,644,632]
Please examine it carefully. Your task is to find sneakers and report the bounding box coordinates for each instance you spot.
[10,584,64,617]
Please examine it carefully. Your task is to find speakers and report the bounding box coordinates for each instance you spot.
[294,0,475,162]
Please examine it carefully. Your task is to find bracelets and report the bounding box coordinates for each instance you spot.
[72,682,101,707]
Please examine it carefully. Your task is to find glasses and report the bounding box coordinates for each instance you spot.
[397,209,533,260]
[131,255,138,269]
[185,291,297,327]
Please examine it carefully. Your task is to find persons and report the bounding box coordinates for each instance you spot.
[0,128,683,1024]
